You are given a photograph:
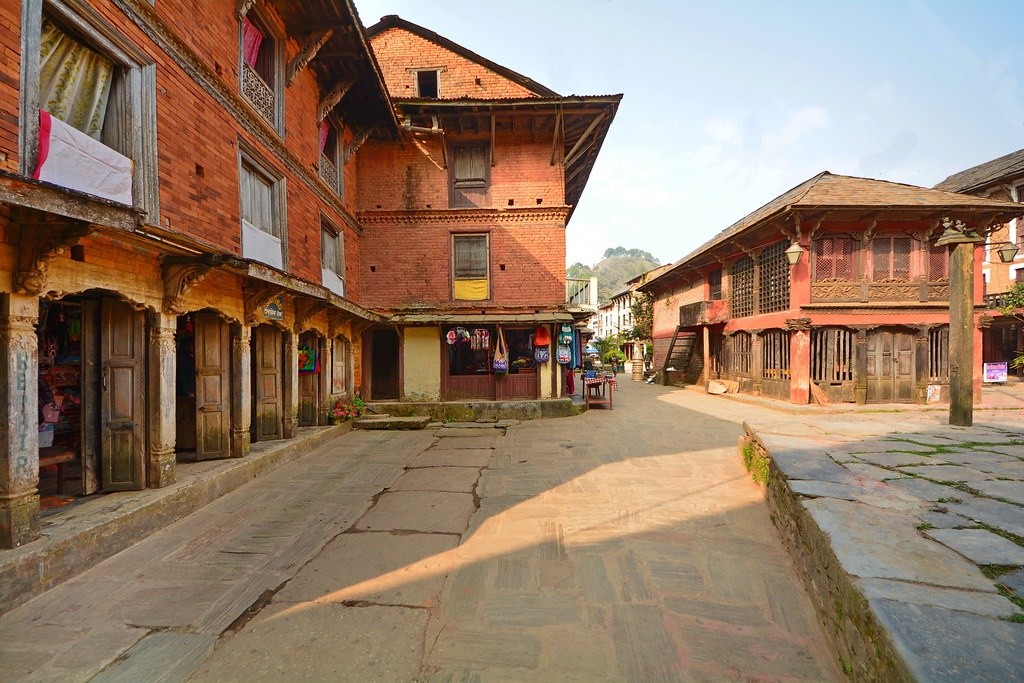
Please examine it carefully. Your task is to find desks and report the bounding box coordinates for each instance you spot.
[581,374,618,410]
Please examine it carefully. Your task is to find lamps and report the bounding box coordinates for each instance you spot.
[784,242,805,264]
[984,241,1022,263]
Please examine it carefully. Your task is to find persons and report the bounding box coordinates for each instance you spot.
[609,352,618,377]
